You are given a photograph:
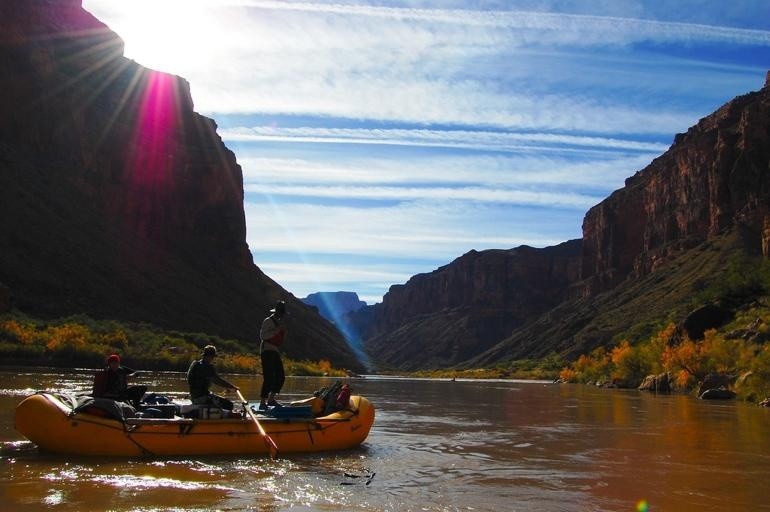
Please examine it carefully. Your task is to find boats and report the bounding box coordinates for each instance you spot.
[12,388,379,460]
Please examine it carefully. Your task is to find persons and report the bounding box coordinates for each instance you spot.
[187,345,240,410]
[260,313,285,410]
[94,354,147,407]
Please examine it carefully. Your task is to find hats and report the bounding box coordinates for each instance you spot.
[108,354,120,363]
[201,344,220,358]
[269,301,290,315]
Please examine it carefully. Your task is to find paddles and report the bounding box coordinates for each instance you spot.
[236,389,278,459]
[127,418,314,425]
